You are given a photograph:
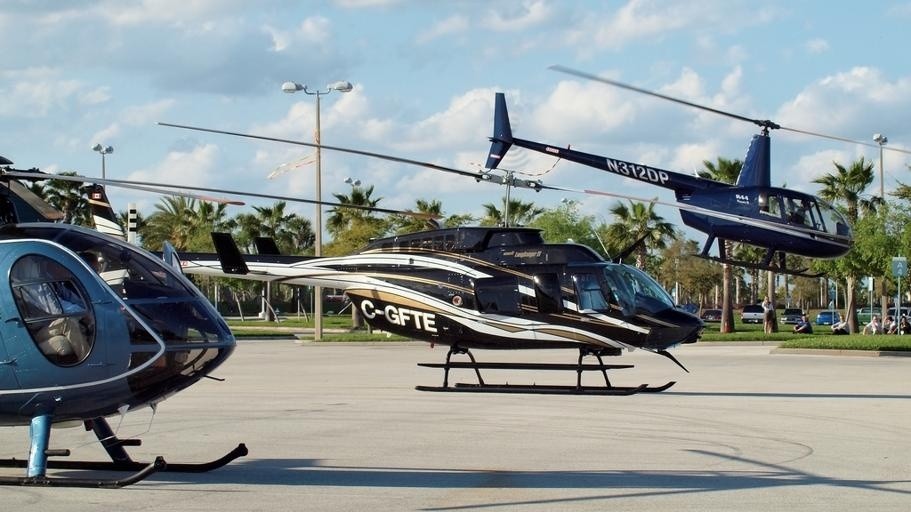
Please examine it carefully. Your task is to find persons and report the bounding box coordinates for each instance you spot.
[581,273,615,310]
[10,254,95,362]
[863,315,911,335]
[794,314,813,334]
[791,199,815,224]
[762,296,775,333]
[831,315,850,335]
[63,252,100,298]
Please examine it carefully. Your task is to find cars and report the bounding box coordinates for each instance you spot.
[701,308,722,323]
[741,302,764,324]
[780,303,911,326]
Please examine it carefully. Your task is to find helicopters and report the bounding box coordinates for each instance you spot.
[79,120,857,395]
[1,164,445,489]
[483,63,911,280]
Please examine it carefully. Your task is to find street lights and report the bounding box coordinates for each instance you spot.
[281,81,354,344]
[873,133,889,211]
[94,142,114,194]
